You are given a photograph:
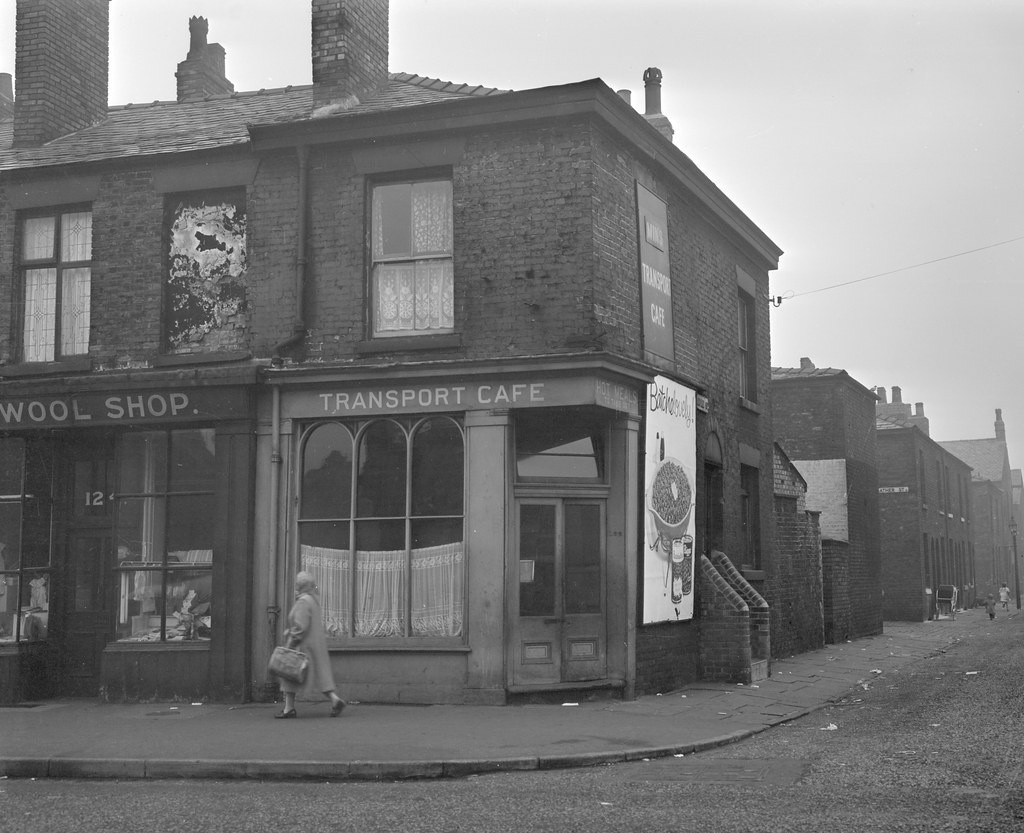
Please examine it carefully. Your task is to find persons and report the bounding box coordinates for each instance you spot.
[268,570,345,718]
[998,582,1011,612]
[983,594,997,620]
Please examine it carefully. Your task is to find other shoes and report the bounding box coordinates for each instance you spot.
[274,709,296,719]
[331,700,345,717]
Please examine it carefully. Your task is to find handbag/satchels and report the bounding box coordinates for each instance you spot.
[267,636,308,683]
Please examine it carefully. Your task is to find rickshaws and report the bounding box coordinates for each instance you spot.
[935,584,958,622]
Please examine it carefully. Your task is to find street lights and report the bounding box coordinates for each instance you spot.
[1009,515,1021,609]
